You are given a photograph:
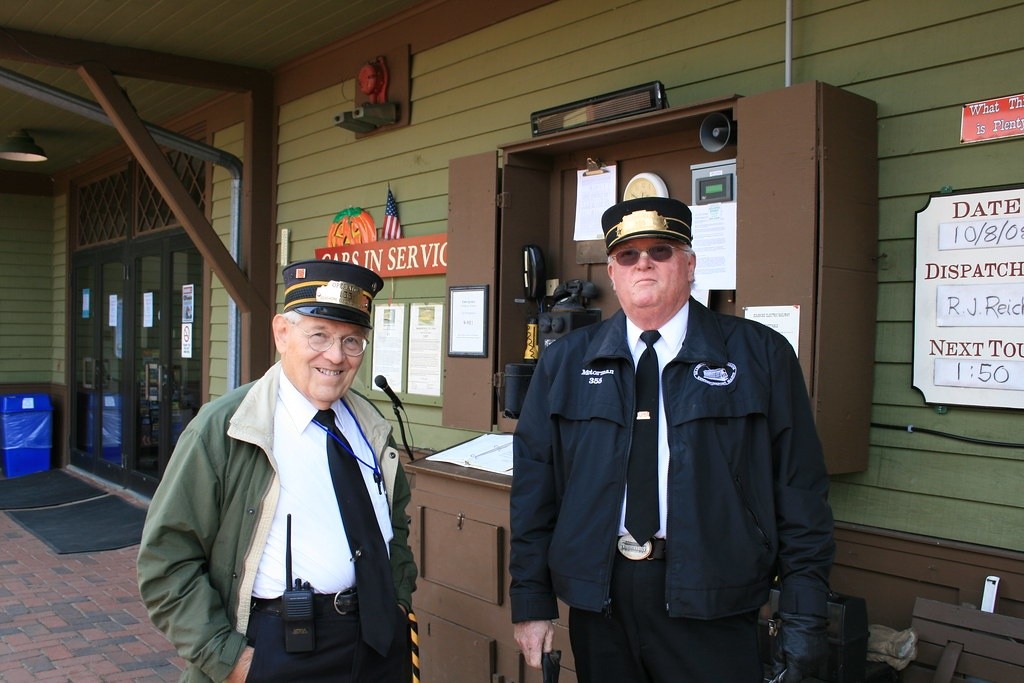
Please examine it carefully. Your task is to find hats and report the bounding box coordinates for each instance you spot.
[282,260,383,329]
[601,197,692,255]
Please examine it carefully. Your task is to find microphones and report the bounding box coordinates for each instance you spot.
[375,375,404,411]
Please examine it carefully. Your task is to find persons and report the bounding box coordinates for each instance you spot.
[509,197,834,683]
[137,259,418,683]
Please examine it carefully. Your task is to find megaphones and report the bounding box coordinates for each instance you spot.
[700,113,737,153]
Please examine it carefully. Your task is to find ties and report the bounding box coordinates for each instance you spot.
[313,410,399,657]
[624,330,661,546]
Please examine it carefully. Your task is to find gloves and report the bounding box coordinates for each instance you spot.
[768,611,828,683]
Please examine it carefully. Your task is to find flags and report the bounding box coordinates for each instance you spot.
[381,183,404,240]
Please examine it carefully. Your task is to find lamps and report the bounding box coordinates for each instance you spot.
[0,128,48,162]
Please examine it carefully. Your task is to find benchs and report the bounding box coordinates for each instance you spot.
[902,597,1024,683]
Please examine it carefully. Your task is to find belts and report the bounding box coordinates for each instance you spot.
[251,592,358,617]
[614,536,665,561]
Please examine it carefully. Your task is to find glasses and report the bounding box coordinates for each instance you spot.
[297,326,369,357]
[612,244,690,266]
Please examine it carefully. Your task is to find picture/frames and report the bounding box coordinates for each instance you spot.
[447,284,490,359]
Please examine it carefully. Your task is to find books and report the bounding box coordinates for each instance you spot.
[426,433,514,476]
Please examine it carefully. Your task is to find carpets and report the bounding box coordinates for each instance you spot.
[0,467,109,510]
[4,494,148,554]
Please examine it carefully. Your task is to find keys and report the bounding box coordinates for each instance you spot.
[373,472,382,495]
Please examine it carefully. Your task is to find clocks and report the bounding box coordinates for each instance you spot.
[623,171,669,202]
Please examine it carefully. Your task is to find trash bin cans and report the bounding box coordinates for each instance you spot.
[0,391,54,477]
[85,394,122,465]
